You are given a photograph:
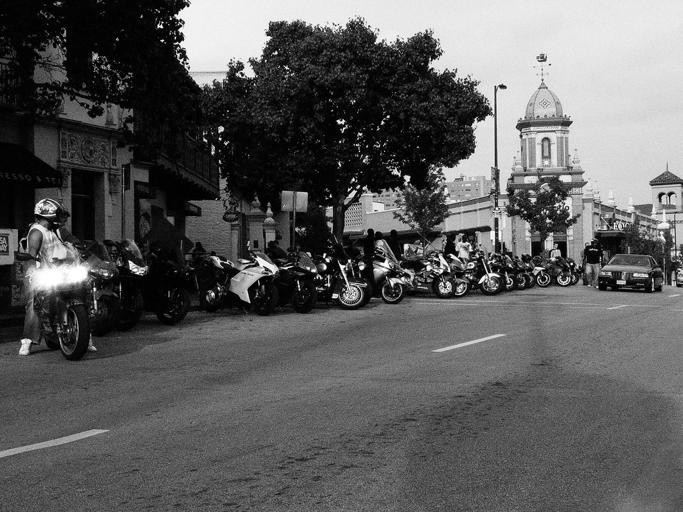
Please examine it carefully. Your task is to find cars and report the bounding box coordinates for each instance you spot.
[674,269,682,288]
[597,254,662,293]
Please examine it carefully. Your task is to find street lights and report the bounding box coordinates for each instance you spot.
[491,81,509,250]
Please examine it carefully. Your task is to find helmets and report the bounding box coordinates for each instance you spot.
[33,198,70,224]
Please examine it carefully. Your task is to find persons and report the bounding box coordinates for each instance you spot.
[18,198,98,356]
[343,226,401,260]
[191,242,224,271]
[455,234,473,265]
[263,239,288,262]
[581,240,603,288]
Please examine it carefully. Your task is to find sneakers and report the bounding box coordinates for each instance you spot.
[88,341,97,352]
[18,338,32,356]
